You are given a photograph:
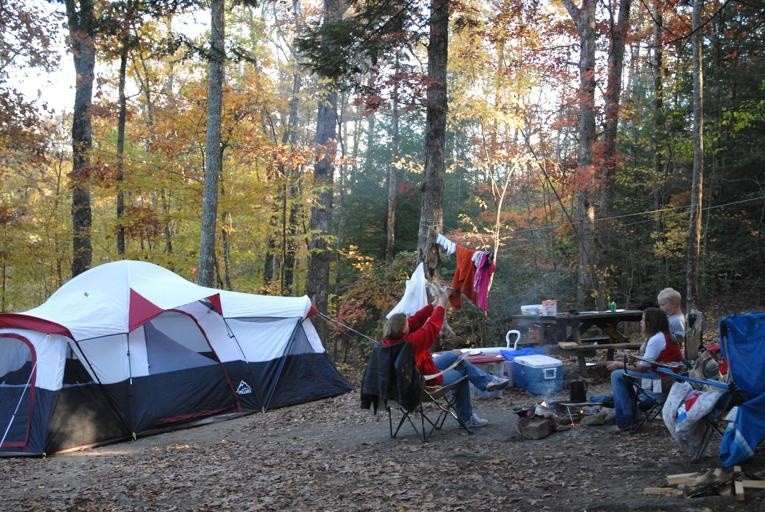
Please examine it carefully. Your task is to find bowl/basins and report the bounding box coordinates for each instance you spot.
[512,406,529,416]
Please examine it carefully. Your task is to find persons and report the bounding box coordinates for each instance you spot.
[383,291,509,428]
[588,286,686,423]
[604,307,685,432]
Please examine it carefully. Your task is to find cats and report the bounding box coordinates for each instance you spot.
[425,275,457,351]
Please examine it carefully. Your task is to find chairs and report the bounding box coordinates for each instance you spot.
[374,341,474,443]
[623,312,764,466]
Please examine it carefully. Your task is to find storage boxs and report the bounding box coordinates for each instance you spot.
[433,347,564,400]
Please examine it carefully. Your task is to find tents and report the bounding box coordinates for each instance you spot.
[0,258,357,459]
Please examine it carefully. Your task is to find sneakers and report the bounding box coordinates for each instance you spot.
[457,414,489,429]
[484,374,510,392]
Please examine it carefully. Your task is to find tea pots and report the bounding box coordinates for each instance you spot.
[569,375,588,403]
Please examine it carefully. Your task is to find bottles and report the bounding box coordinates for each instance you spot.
[609,300,617,312]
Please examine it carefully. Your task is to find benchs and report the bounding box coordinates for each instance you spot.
[508,337,628,352]
[571,343,642,378]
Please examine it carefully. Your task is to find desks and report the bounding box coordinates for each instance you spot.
[511,310,644,342]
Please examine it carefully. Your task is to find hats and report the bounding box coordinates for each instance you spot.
[696,350,720,379]
